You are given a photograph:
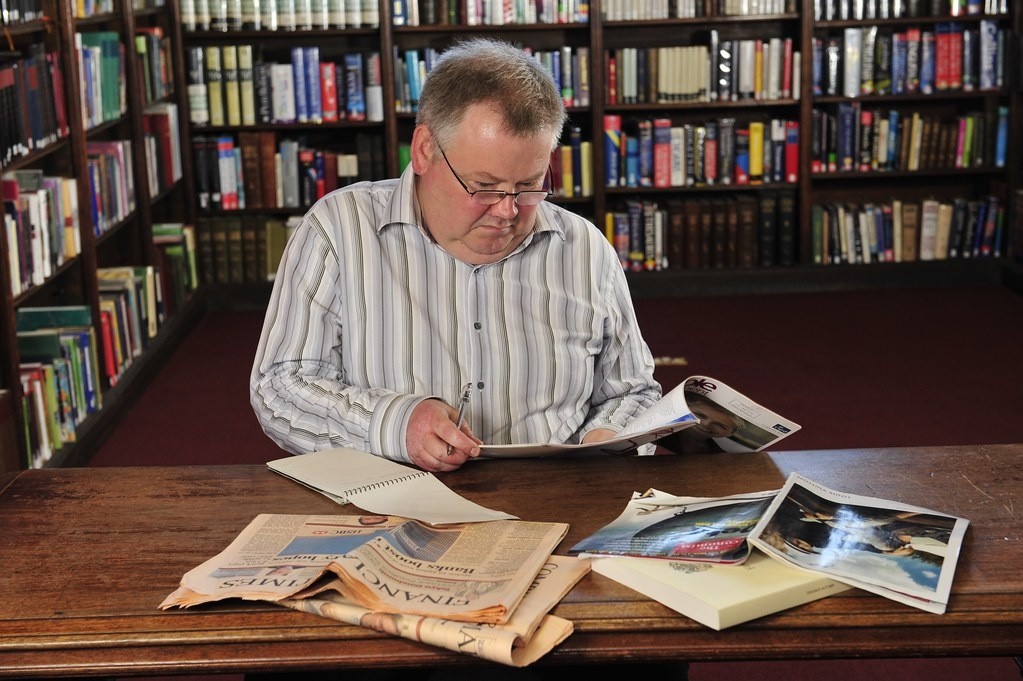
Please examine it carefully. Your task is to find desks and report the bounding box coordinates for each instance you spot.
[0,443,1023,680]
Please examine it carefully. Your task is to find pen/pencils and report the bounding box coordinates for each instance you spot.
[447,383,473,456]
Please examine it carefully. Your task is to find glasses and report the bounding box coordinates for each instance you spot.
[434,134,554,205]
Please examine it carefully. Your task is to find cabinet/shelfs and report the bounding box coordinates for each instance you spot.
[185,0,1023,305]
[1,0,206,467]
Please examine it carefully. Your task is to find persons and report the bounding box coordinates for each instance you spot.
[267,565,306,576]
[359,516,388,525]
[650,394,745,454]
[777,512,913,556]
[249,37,662,472]
[304,598,404,636]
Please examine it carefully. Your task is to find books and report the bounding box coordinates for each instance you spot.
[602,114,797,275]
[179,1,379,31]
[567,471,971,631]
[810,101,1009,265]
[477,375,802,459]
[266,446,520,526]
[600,0,800,105]
[191,132,388,210]
[0,0,200,469]
[195,215,303,287]
[811,0,1006,98]
[186,41,385,127]
[391,0,596,198]
